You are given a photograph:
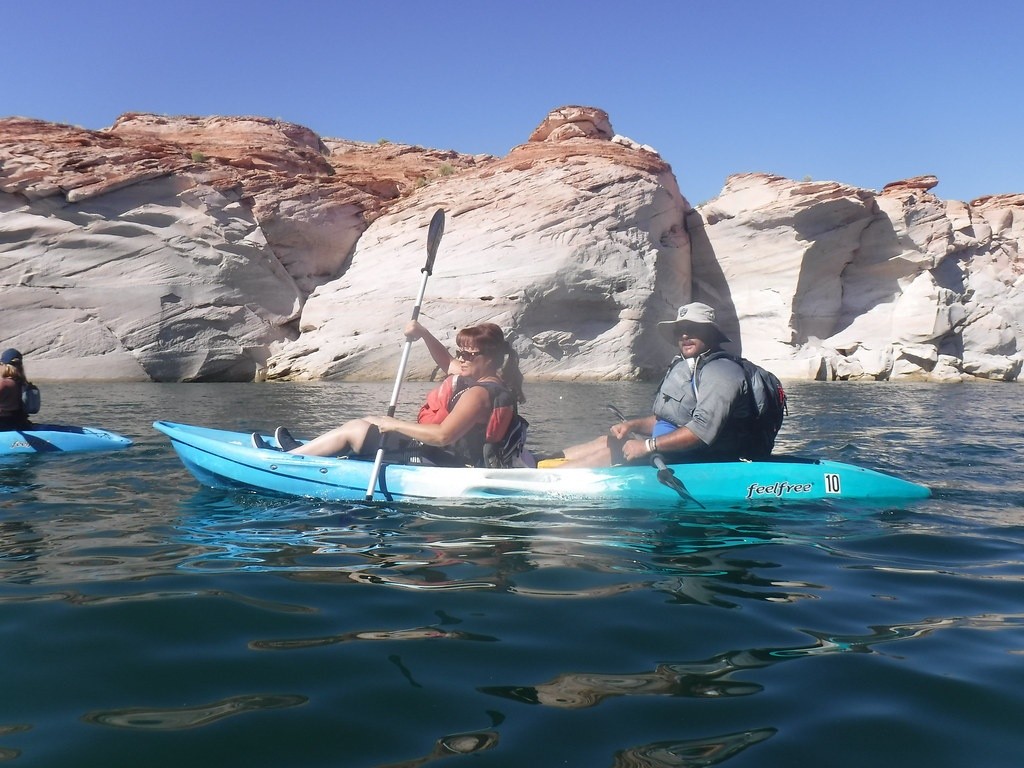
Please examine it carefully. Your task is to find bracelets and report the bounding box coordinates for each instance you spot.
[652,437,657,451]
[645,438,652,454]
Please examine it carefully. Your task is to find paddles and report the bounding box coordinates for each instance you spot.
[604,405,695,499]
[364,206,448,502]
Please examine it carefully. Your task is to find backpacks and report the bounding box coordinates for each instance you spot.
[659,348,789,456]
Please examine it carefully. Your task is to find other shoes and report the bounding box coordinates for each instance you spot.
[483,443,509,469]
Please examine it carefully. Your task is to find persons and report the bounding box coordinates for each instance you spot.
[250,319,526,468]
[533,302,750,469]
[0,348,28,431]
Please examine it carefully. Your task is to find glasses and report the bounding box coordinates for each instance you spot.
[456,349,483,360]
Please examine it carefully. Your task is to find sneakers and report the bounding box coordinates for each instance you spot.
[275,426,304,452]
[251,431,279,448]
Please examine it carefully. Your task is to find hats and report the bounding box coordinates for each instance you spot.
[0,348,22,367]
[656,301,733,347]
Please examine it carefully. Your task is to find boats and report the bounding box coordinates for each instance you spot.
[155,419,933,505]
[0,427,135,455]
